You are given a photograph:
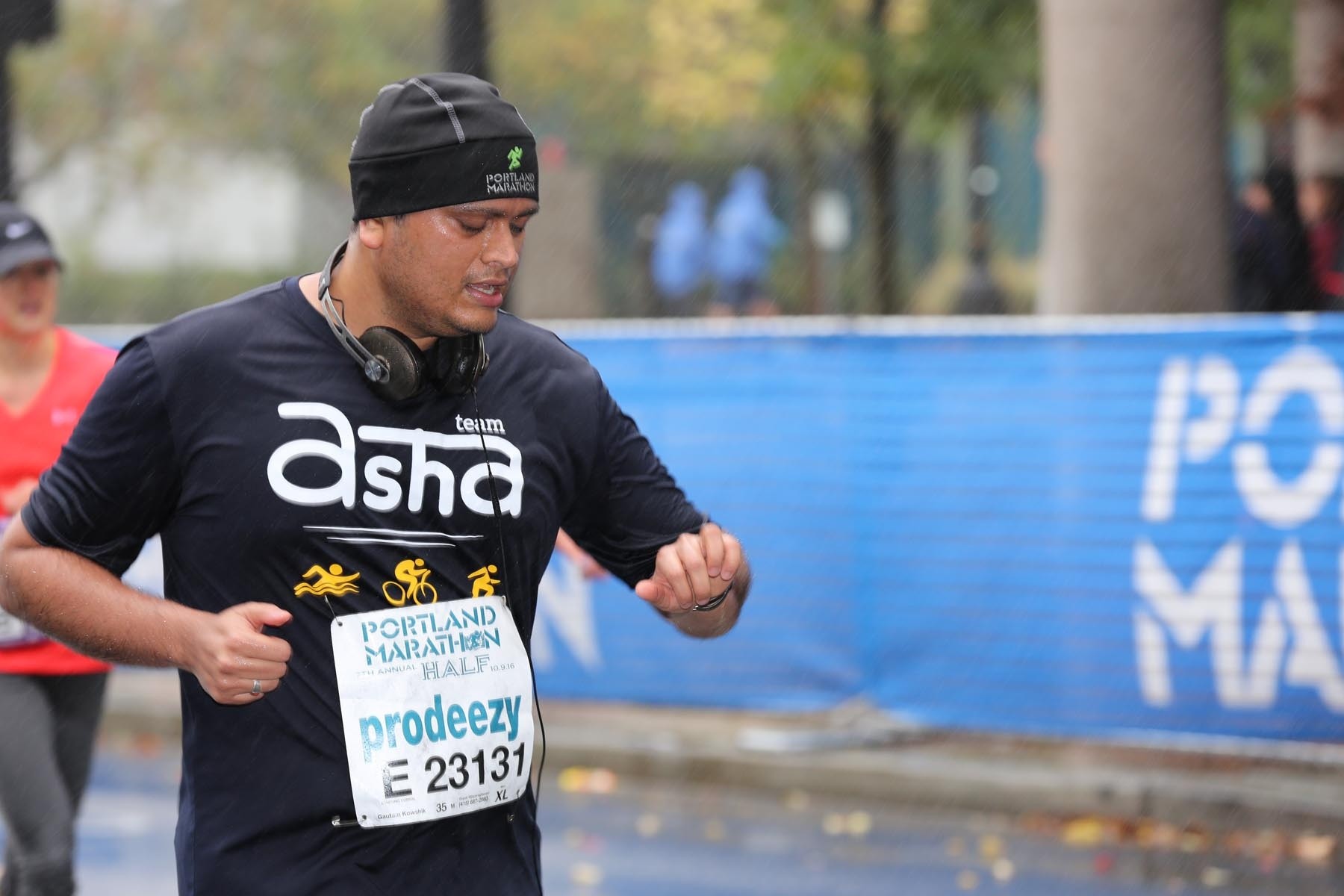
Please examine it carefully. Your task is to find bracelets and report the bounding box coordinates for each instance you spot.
[692,582,736,613]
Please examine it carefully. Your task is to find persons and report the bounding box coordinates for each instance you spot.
[0,73,753,896]
[1229,163,1343,312]
[656,164,801,318]
[0,203,115,896]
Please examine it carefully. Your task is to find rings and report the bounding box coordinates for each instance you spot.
[252,680,261,694]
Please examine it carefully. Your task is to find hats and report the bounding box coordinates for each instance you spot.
[0,201,62,276]
[348,72,539,223]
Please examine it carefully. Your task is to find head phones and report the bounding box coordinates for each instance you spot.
[319,239,496,407]
